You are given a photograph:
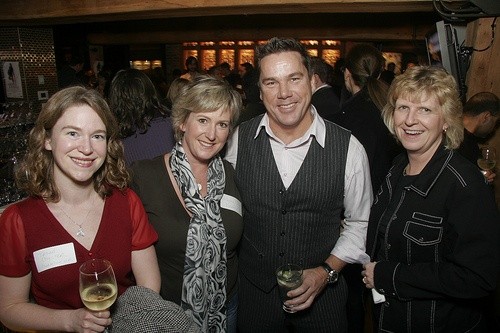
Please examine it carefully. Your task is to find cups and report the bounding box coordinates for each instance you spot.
[275,263,304,313]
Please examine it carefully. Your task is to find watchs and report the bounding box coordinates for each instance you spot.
[321,263,338,284]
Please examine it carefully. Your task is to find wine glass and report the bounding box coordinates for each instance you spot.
[79,258,118,332]
[477,146,497,184]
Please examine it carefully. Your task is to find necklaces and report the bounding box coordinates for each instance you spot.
[197,169,207,191]
[62,209,90,236]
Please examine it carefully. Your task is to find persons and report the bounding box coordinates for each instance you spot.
[107,45,499,185]
[362,65,500,333]
[1,86,162,333]
[224,39,373,333]
[126,74,243,333]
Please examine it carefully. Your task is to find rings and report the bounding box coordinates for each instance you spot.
[363,277,367,283]
